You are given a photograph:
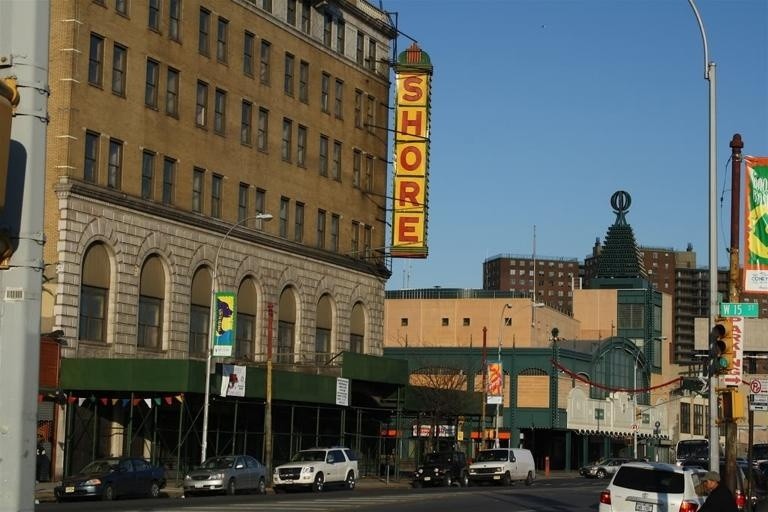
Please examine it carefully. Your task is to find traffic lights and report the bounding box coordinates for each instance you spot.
[712,317,734,371]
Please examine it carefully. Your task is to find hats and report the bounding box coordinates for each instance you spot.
[701,471,720,483]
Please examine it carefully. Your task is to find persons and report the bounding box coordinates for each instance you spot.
[695,469,738,511]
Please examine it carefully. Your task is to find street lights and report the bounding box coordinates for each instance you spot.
[631,333,669,460]
[200,211,274,468]
[495,301,546,447]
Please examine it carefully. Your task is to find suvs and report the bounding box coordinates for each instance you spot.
[413,450,470,486]
[272,446,359,493]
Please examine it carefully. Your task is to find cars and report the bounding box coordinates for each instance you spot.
[54,454,166,500]
[580,457,630,480]
[182,455,269,494]
[681,456,766,497]
[598,459,710,512]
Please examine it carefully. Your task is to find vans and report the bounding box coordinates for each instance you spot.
[675,439,710,469]
[467,446,536,486]
